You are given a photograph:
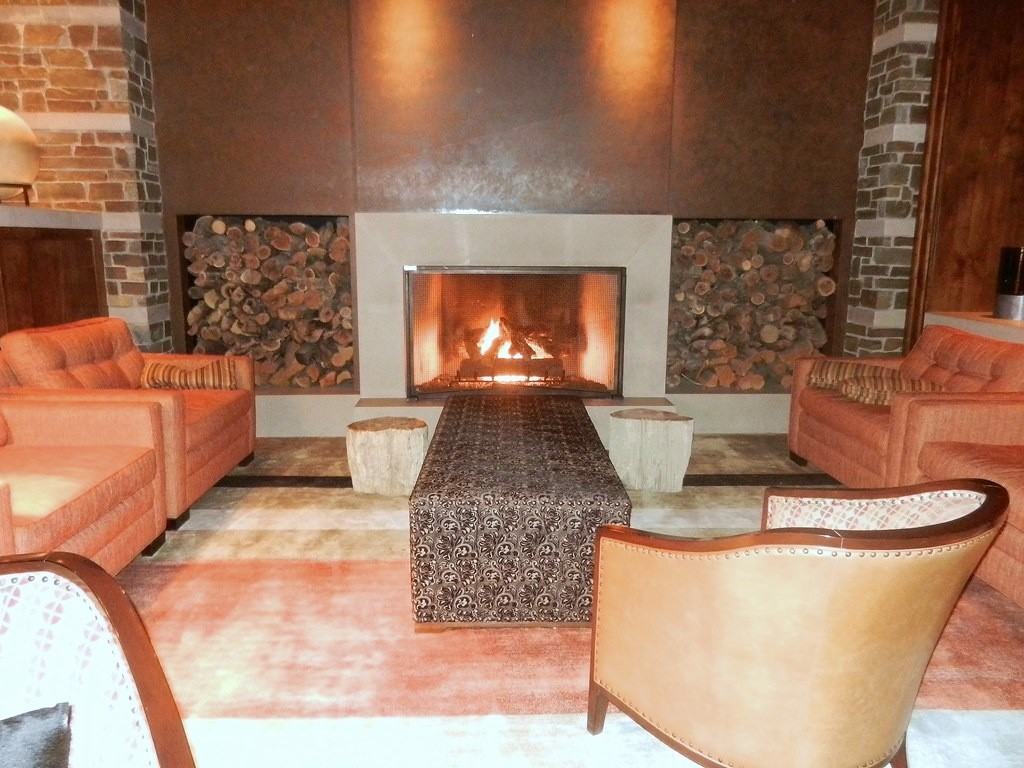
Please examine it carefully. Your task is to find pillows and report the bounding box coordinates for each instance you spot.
[140,357,238,392]
[807,358,903,392]
[840,374,946,406]
[0,703,74,768]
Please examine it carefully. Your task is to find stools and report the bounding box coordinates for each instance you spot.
[608,408,695,493]
[346,416,428,497]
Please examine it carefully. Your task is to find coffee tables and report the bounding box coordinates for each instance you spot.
[409,393,631,629]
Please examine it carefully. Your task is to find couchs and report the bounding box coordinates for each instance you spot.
[0,396,167,576]
[789,323,1024,488]
[899,399,1024,608]
[2,316,259,531]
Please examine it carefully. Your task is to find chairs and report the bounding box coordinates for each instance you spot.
[584,477,1010,768]
[0,552,199,768]
